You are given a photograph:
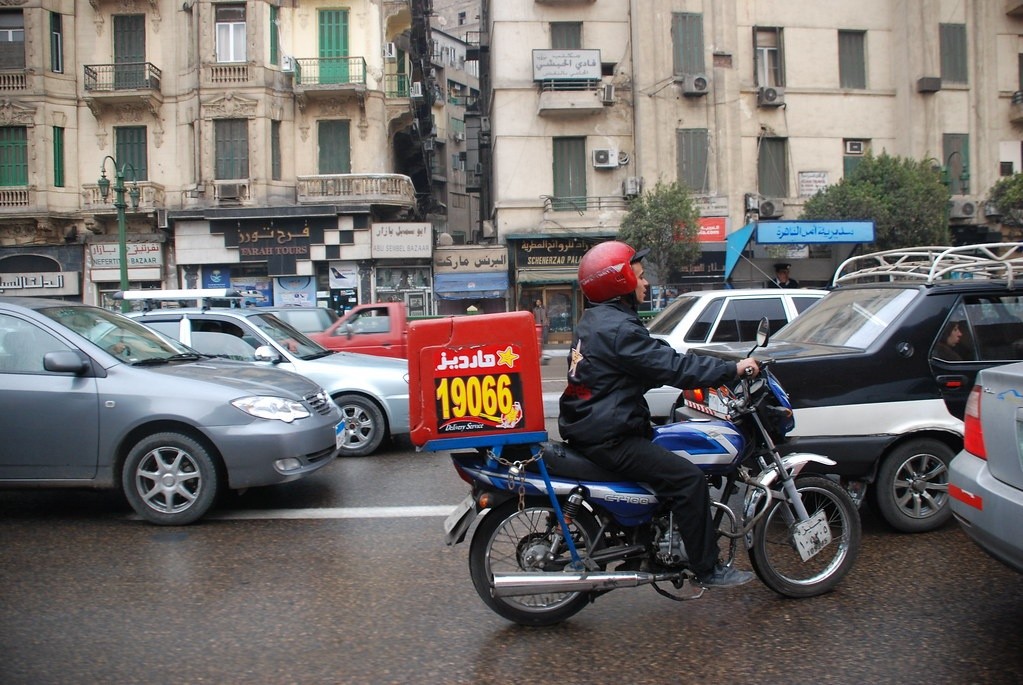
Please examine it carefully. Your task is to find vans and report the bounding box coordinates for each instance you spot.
[240,306,337,353]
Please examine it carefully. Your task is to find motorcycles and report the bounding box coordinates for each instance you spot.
[405,310,861,632]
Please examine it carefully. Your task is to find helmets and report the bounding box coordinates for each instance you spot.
[579,243,638,303]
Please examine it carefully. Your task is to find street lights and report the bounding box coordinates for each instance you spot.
[97,155,140,358]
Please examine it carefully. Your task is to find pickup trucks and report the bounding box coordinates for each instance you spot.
[276,302,543,363]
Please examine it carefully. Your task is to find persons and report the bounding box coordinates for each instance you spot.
[557,243,758,590]
[533,299,550,344]
[766,261,801,289]
[933,315,965,361]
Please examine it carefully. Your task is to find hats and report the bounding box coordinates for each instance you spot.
[774,263,791,271]
[631,247,651,263]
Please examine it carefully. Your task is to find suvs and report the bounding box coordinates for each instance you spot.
[668,242,1023,535]
[34,290,411,459]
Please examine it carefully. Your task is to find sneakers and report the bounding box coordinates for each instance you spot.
[702,562,755,588]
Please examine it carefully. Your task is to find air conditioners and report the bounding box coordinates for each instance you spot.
[603,85,615,103]
[218,184,239,198]
[622,178,639,200]
[758,199,783,217]
[424,139,433,150]
[457,132,466,140]
[758,87,784,106]
[413,82,421,95]
[949,198,976,219]
[282,55,295,71]
[386,42,397,63]
[480,117,490,132]
[592,149,619,167]
[682,75,709,97]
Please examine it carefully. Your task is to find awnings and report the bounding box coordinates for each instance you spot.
[435,271,510,301]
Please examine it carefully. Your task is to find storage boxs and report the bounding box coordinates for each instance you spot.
[407,312,545,445]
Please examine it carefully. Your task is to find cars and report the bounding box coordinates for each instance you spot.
[1,295,348,526]
[944,363,1023,578]
[640,288,885,427]
